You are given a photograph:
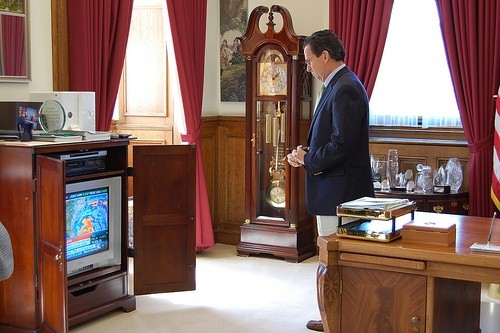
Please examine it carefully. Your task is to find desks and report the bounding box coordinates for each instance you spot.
[374,190,469,216]
[316,212,500,333]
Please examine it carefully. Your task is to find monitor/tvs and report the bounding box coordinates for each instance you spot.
[64,175,124,276]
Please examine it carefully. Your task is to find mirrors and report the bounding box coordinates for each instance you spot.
[0,0,33,84]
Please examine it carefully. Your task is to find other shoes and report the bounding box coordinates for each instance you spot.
[306,320,325,332]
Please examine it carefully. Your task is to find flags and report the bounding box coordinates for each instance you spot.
[490,88,500,214]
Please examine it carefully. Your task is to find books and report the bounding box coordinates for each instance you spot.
[31,132,82,143]
[63,129,111,141]
[340,196,408,211]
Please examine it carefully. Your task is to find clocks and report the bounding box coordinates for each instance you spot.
[261,65,287,94]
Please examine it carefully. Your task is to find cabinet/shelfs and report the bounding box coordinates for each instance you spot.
[0,138,197,333]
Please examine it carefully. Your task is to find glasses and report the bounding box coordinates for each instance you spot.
[305,50,323,67]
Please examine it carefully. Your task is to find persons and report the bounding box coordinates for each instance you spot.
[287,30,375,331]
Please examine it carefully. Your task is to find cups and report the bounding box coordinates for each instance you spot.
[370,149,463,194]
[17,121,33,141]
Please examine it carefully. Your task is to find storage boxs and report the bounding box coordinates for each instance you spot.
[402,220,456,247]
[336,197,417,243]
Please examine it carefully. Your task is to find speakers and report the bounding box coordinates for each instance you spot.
[77,91,96,131]
[43,92,77,131]
